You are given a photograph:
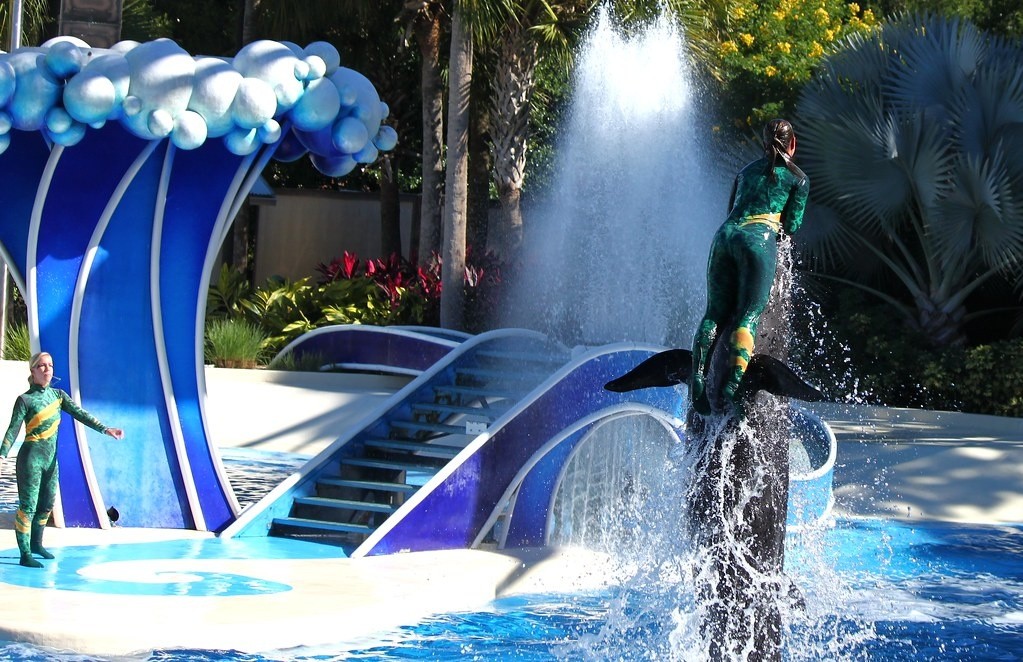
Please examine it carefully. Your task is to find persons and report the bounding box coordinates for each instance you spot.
[0,351,125,569]
[692,120,809,415]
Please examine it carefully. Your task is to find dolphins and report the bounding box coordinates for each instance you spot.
[601,228,829,662]
